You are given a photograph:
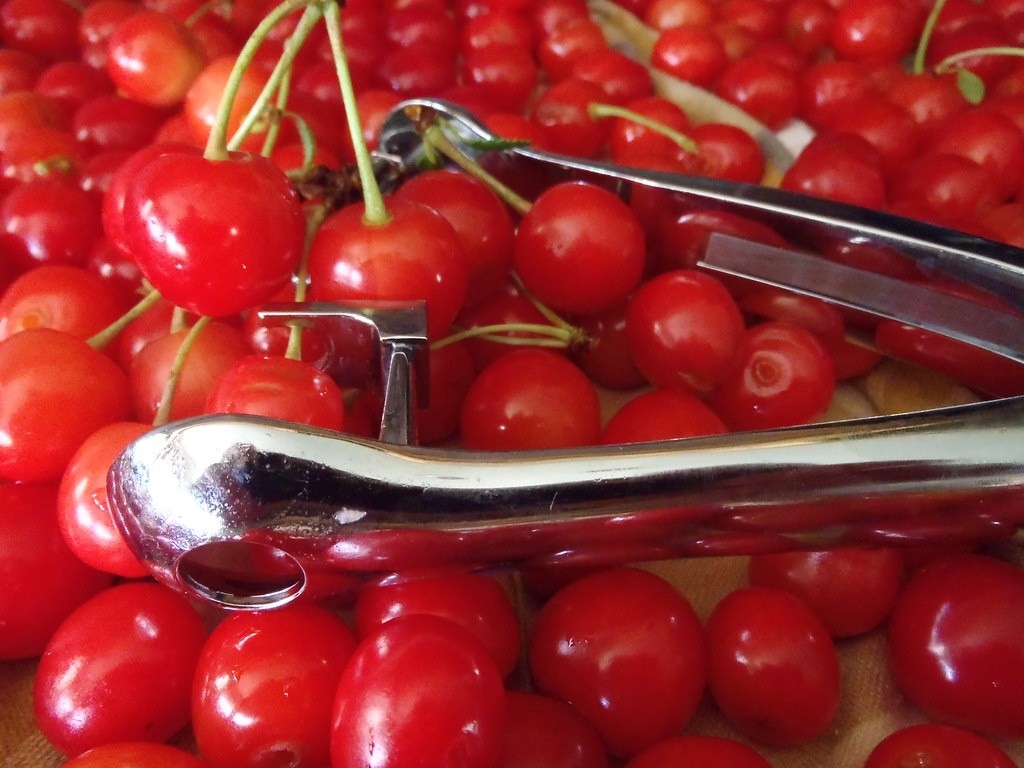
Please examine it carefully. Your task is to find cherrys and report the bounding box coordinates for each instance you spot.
[0,0,1024,768]
